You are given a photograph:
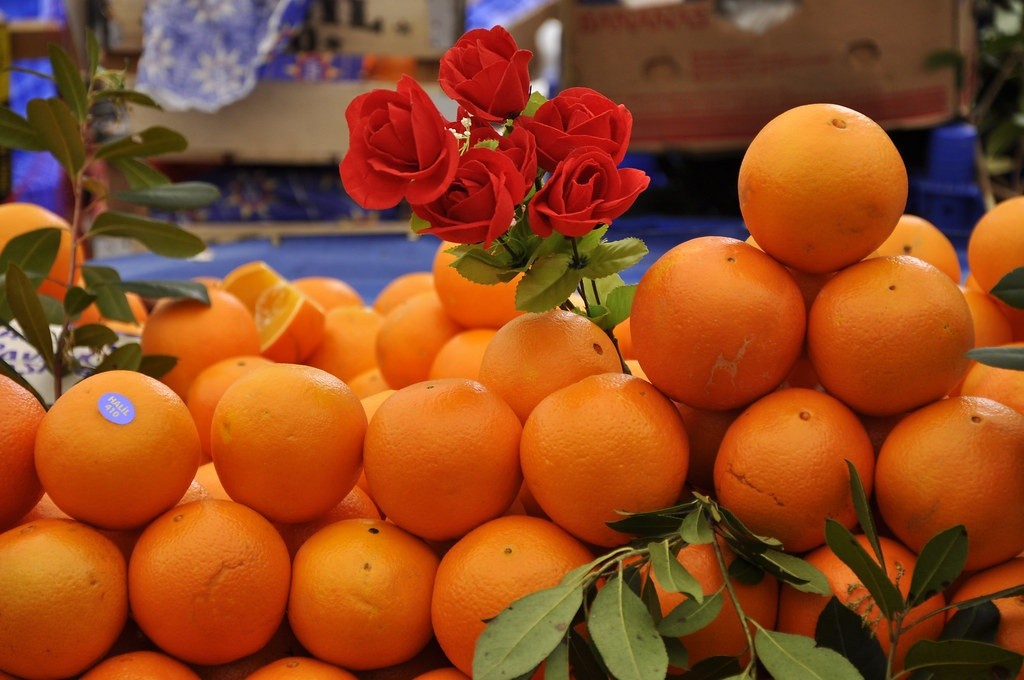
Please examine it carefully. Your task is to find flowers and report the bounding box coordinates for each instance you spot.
[340,24,651,378]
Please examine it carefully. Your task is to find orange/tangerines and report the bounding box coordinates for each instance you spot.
[0,99,1024,680]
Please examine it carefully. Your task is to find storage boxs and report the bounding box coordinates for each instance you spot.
[567,0,972,151]
